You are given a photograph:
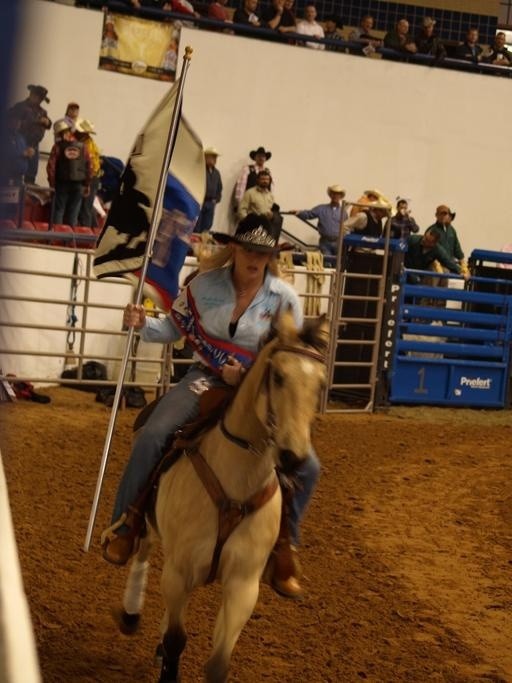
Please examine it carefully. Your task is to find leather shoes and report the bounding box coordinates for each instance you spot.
[103,533,133,565]
[271,571,304,598]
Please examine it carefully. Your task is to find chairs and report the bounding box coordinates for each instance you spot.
[1,182,226,261]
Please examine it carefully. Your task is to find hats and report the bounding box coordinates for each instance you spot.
[365,191,393,217]
[212,214,295,253]
[205,147,221,155]
[328,185,345,198]
[436,206,455,220]
[53,120,72,136]
[250,147,272,160]
[74,119,98,135]
[27,84,50,103]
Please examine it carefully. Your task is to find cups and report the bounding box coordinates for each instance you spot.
[496,53,503,61]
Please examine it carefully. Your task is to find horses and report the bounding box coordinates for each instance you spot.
[118,302,331,683]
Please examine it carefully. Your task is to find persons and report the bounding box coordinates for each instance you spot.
[101,213,321,598]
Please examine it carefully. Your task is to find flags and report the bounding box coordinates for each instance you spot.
[94,77,207,311]
